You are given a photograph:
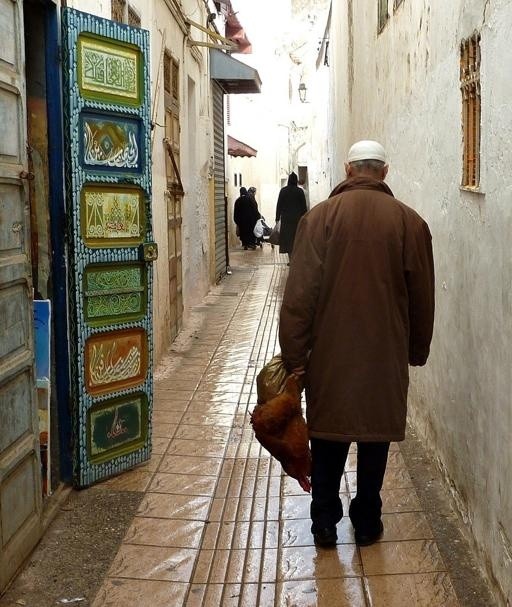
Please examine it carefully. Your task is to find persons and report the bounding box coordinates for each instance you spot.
[297,179,310,211]
[234,186,264,250]
[277,139,435,549]
[275,172,308,267]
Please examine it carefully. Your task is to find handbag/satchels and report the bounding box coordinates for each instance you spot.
[270,231,279,244]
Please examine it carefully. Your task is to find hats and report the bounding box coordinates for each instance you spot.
[345,140,388,165]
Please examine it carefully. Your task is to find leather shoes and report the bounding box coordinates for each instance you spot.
[313,525,337,546]
[355,522,384,545]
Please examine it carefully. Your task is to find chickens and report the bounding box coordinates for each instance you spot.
[248,375,315,494]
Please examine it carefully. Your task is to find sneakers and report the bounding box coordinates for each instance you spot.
[244,245,255,249]
